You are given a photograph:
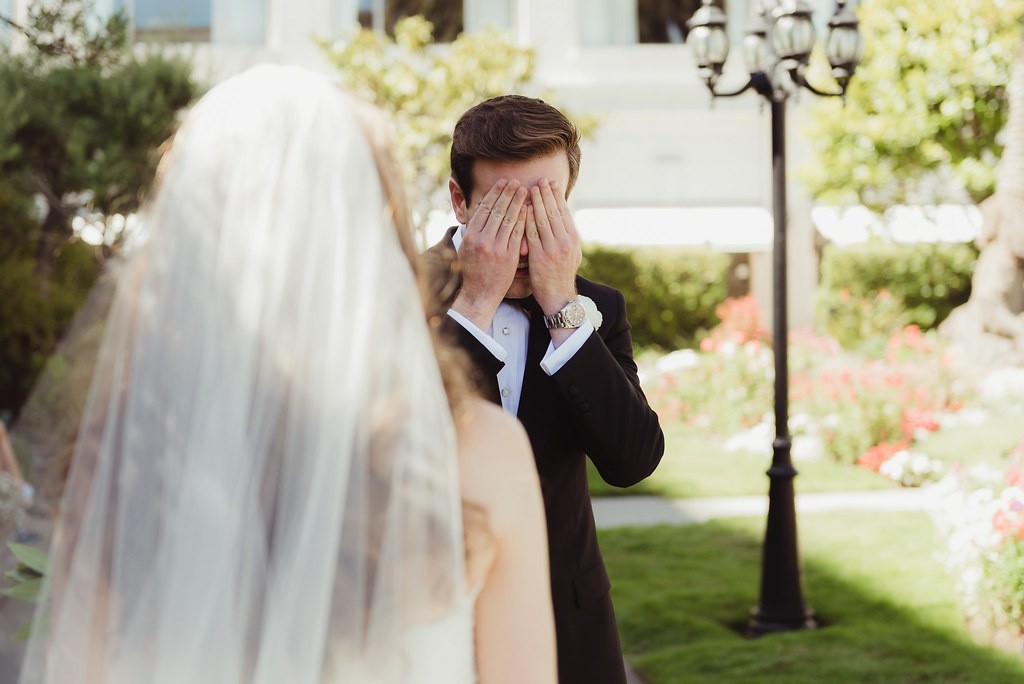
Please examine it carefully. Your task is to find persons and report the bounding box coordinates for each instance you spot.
[42,66,557,683]
[410,93,666,683]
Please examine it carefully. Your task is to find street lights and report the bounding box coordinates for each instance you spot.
[687,0,861,647]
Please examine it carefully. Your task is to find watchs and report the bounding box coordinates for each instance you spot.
[541,299,585,330]
